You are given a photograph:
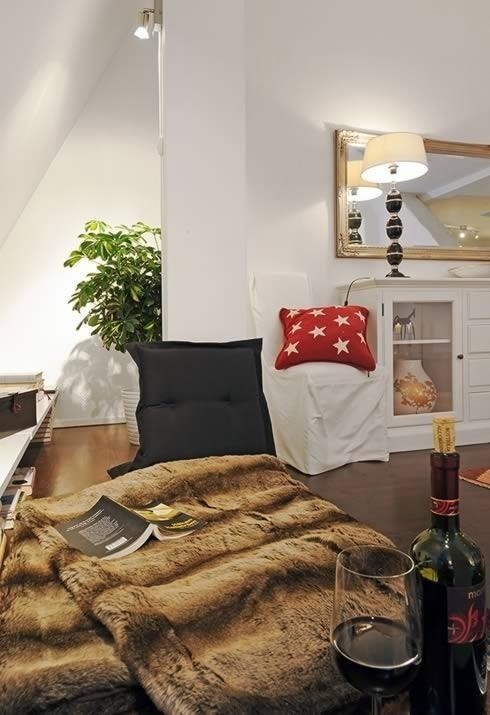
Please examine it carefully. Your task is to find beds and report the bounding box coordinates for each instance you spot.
[0,451,421,714]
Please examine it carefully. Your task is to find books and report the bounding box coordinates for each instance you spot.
[6,467,40,495]
[0,487,23,530]
[51,493,208,563]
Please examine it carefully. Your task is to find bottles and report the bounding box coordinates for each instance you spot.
[410,452,490,713]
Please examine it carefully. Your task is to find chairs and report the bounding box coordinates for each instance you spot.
[247,267,392,479]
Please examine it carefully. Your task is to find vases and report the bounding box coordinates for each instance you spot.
[393,359,439,415]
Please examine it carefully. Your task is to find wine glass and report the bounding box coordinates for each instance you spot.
[329,541,422,715]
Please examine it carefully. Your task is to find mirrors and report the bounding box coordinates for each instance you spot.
[334,124,489,263]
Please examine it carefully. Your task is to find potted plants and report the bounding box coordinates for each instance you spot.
[62,215,164,446]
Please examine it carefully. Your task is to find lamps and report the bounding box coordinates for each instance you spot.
[134,0,163,41]
[358,131,434,279]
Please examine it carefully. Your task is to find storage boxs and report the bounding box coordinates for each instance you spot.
[0,386,39,435]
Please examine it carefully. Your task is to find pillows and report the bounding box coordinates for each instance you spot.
[106,335,279,481]
[346,158,383,246]
[275,303,378,374]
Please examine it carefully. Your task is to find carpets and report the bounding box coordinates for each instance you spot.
[458,465,489,490]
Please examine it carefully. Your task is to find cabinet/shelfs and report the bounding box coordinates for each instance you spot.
[339,274,490,452]
